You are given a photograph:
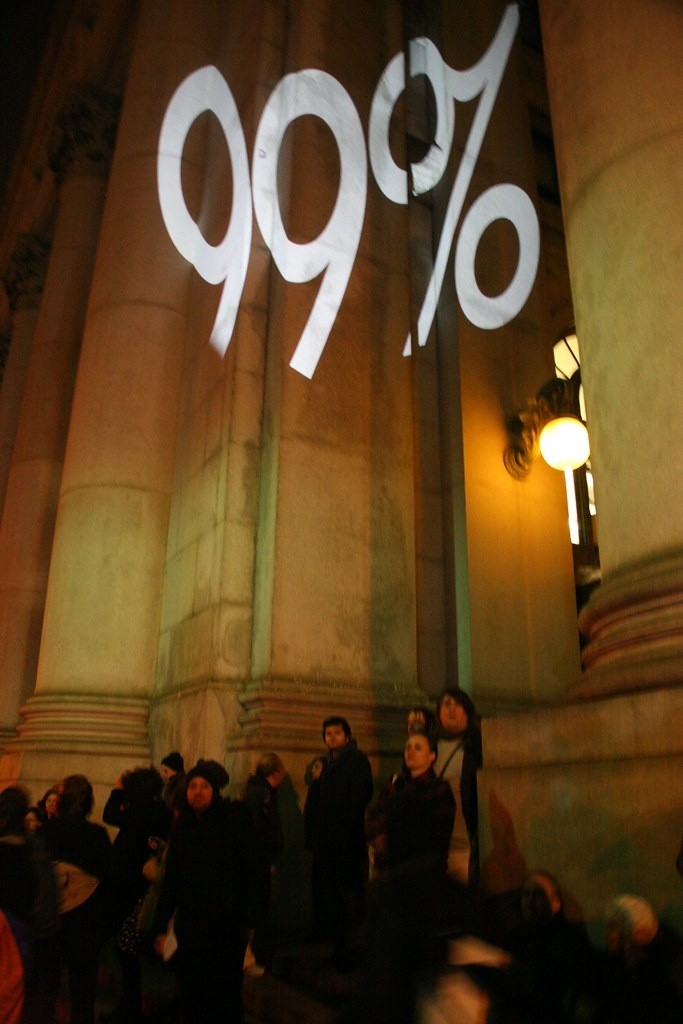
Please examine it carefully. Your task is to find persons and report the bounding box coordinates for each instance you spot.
[416,871,682,1024]
[0,686,483,1024]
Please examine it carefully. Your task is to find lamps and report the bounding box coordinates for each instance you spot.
[504,370,590,482]
